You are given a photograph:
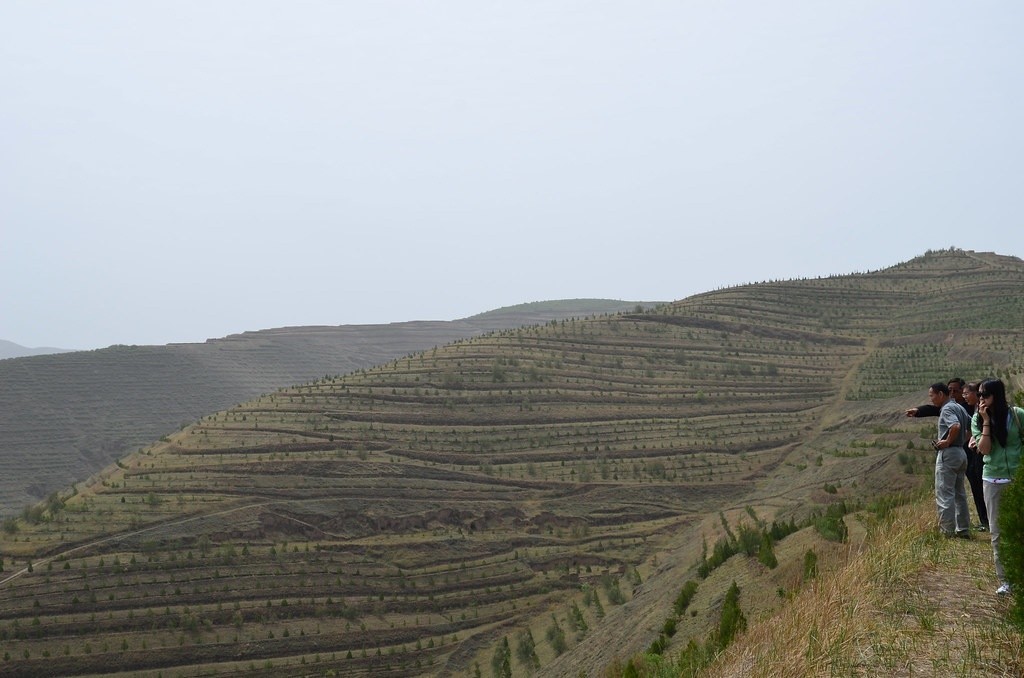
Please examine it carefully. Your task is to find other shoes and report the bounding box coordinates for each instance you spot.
[957,530,970,540]
[996,580,1010,594]
[978,526,989,532]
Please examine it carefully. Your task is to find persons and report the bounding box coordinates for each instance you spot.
[905,378,990,538]
[971,378,1024,594]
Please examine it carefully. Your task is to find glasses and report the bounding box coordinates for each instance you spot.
[976,391,992,398]
[932,440,939,450]
[962,392,974,398]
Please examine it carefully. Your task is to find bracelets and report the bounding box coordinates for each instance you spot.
[981,433,990,436]
[983,424,989,426]
